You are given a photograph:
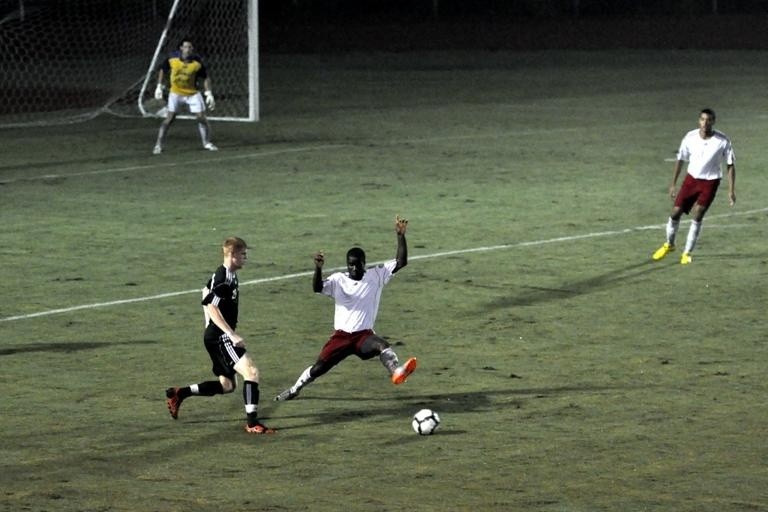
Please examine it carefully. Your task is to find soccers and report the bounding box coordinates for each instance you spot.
[411,409,440,435]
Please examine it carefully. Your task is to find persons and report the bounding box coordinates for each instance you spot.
[166,237,276,434]
[152,39,220,155]
[273,216,417,403]
[653,109,736,263]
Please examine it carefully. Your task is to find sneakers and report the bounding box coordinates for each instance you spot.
[273,388,298,401]
[245,421,277,435]
[390,357,417,386]
[152,145,163,155]
[680,250,694,266]
[652,240,676,261]
[164,386,185,420]
[203,141,219,151]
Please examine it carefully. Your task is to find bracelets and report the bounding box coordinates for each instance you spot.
[225,331,235,338]
